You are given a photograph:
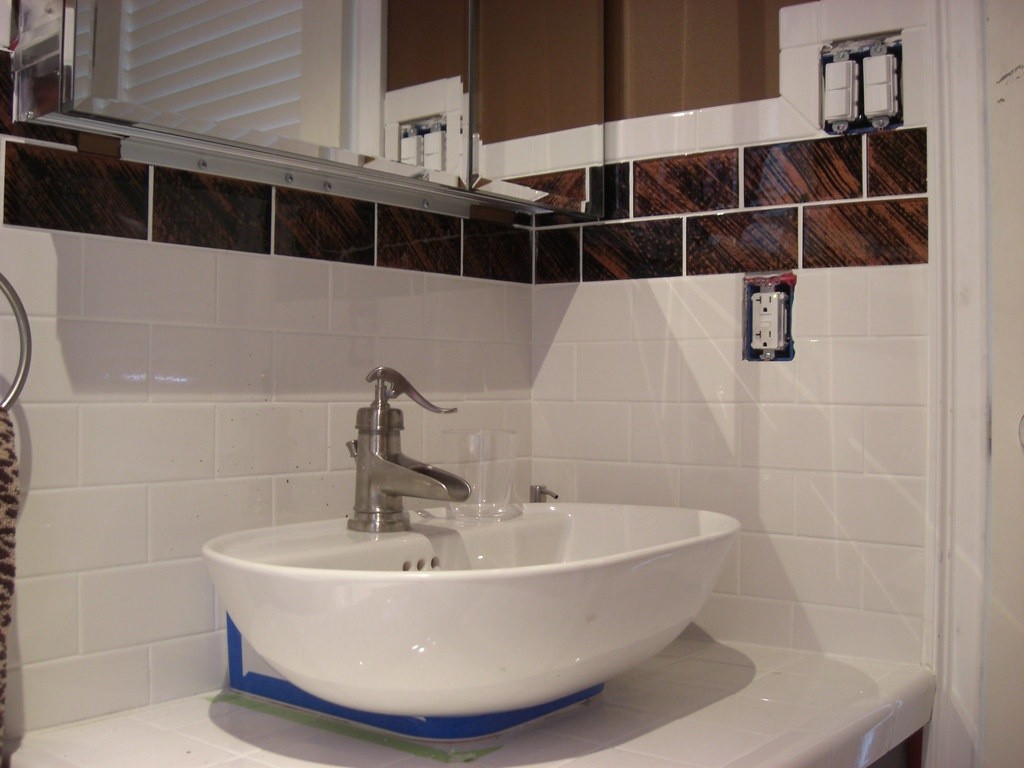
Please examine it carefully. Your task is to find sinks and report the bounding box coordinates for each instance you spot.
[201,500,743,718]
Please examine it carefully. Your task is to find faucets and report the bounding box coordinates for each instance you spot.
[346,367,472,533]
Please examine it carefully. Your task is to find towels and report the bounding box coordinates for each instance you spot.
[0,405,20,740]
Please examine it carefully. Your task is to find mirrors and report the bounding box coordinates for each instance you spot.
[13,0,605,229]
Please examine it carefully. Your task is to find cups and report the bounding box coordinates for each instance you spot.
[442,429,524,523]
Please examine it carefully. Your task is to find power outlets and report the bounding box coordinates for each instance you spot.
[743,276,795,363]
